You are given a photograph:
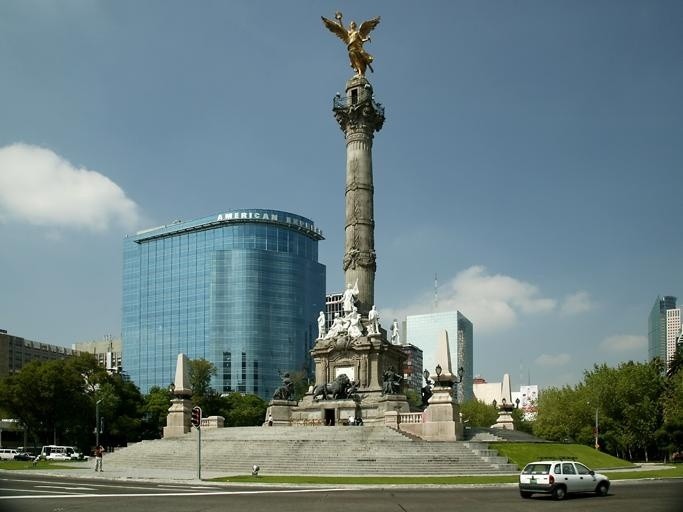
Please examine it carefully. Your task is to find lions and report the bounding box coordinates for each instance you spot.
[312,373,350,401]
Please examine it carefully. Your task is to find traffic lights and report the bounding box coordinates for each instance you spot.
[190,405,200,427]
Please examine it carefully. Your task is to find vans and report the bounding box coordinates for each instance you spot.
[0,448,24,461]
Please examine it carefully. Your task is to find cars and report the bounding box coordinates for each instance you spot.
[23,452,42,462]
[82,455,89,461]
[518,460,611,499]
[46,454,72,462]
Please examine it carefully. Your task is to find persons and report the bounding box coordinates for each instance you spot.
[272,372,294,400]
[348,415,354,425]
[383,366,399,394]
[356,416,363,426]
[338,276,360,317]
[267,414,273,426]
[334,10,370,79]
[94,445,105,472]
[316,305,400,346]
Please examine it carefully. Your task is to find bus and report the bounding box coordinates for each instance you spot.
[16,446,42,458]
[41,444,84,460]
